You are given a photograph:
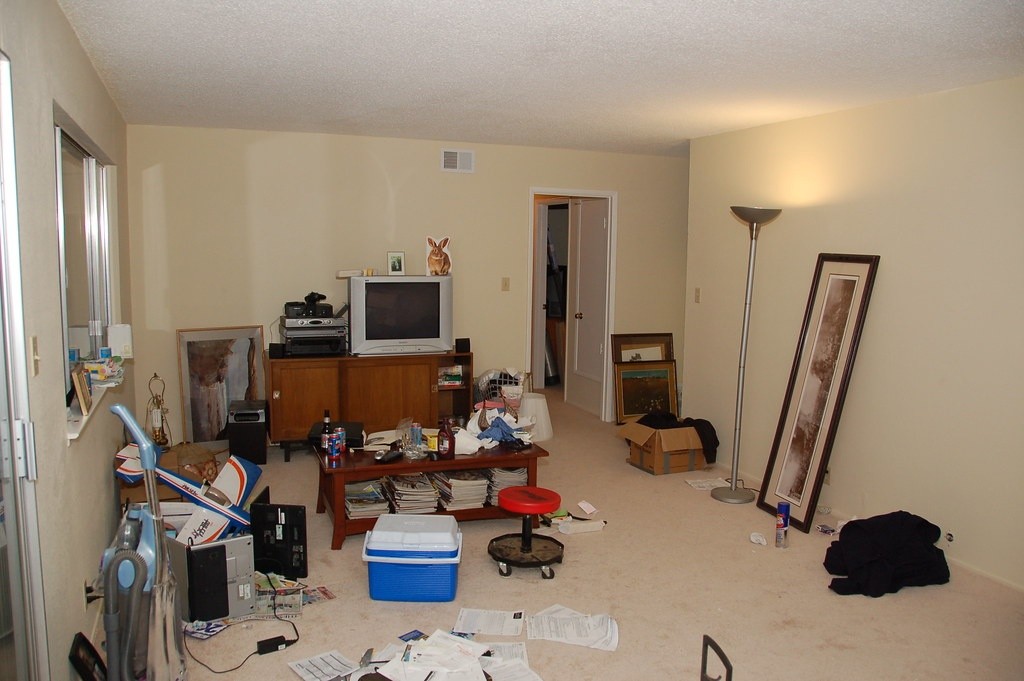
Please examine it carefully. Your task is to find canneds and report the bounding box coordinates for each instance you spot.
[410,423,423,446]
[328,427,346,460]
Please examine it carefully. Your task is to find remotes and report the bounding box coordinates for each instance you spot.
[374,449,404,463]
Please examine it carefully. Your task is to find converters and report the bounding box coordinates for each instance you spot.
[257,636,286,654]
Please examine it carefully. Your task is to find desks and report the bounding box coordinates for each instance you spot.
[307,437,550,553]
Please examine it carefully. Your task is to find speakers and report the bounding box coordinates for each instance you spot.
[455,338,471,353]
[269,343,285,359]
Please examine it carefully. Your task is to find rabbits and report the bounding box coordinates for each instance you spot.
[427,237,451,276]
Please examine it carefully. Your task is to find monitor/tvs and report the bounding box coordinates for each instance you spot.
[350,276,453,356]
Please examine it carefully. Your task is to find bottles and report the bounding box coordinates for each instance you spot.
[321,408,333,452]
[438,418,455,461]
[777,503,790,550]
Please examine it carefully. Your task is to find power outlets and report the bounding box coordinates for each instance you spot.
[823,467,831,486]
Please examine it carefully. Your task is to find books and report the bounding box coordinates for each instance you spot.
[364,430,397,451]
[344,467,528,520]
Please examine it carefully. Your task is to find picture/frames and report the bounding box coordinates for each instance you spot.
[612,359,680,427]
[756,248,881,533]
[386,250,408,275]
[172,324,268,449]
[71,366,93,417]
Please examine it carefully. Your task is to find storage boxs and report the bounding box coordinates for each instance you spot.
[616,412,708,476]
[360,510,465,604]
[228,421,266,466]
[610,332,676,362]
[116,445,183,503]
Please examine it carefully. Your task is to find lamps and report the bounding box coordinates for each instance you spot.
[709,204,781,504]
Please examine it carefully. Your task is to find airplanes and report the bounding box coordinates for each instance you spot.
[114,438,262,545]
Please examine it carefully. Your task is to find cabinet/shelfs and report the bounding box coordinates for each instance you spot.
[265,347,474,464]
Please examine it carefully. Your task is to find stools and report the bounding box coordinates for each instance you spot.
[485,484,570,581]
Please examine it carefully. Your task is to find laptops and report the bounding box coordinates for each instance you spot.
[167,535,256,624]
[249,485,309,578]
